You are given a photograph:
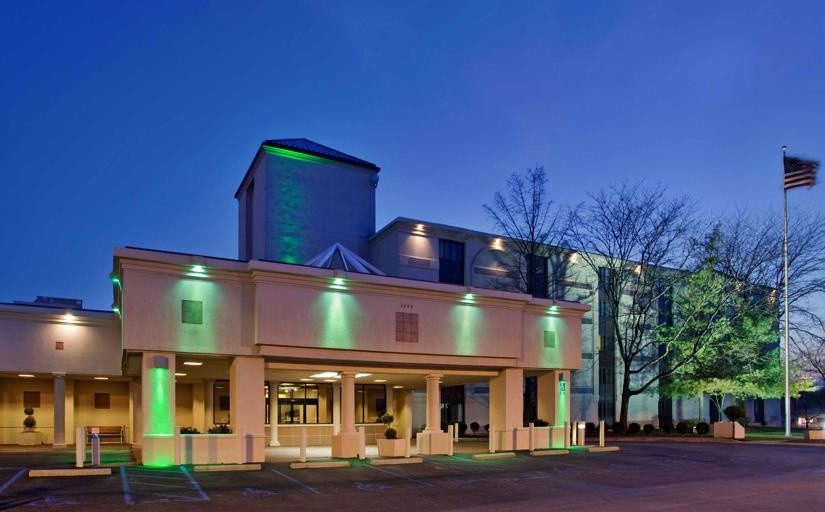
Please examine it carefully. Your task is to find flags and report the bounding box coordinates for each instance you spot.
[784,155,819,191]
